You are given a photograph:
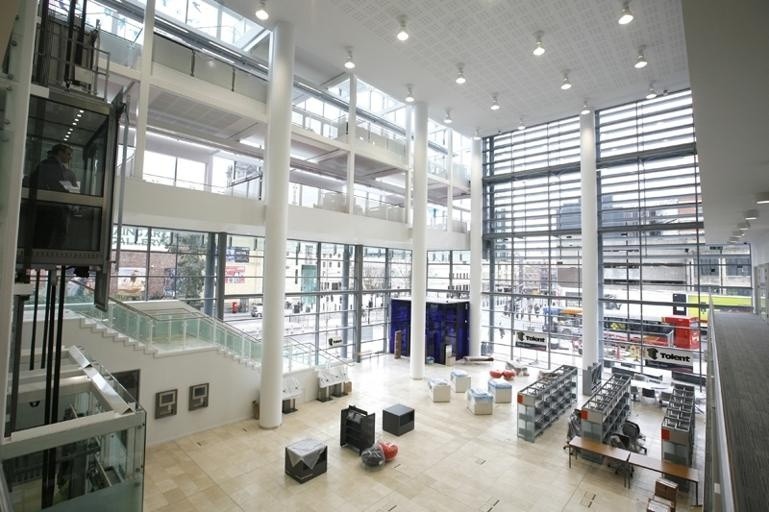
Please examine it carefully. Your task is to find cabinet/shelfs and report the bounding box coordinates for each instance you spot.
[660,384,695,493]
[581,372,631,465]
[517,365,578,443]
[340,405,375,456]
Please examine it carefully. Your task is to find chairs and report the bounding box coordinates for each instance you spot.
[607,420,647,478]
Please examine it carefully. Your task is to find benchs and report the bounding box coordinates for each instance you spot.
[464,356,495,364]
[505,360,527,376]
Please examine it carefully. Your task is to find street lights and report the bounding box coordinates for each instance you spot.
[567,242,581,307]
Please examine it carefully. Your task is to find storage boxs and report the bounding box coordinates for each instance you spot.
[646,479,679,512]
[427,377,451,402]
[466,388,493,415]
[488,378,512,403]
[449,369,471,393]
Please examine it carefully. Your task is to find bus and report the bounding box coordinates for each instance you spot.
[538,289,700,366]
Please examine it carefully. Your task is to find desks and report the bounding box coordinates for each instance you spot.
[628,452,699,507]
[569,436,630,487]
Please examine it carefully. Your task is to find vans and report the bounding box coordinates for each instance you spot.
[251,299,293,319]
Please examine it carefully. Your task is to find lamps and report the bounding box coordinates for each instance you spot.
[256,0,658,143]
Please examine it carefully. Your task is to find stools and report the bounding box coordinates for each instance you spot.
[285,438,327,484]
[383,403,414,436]
